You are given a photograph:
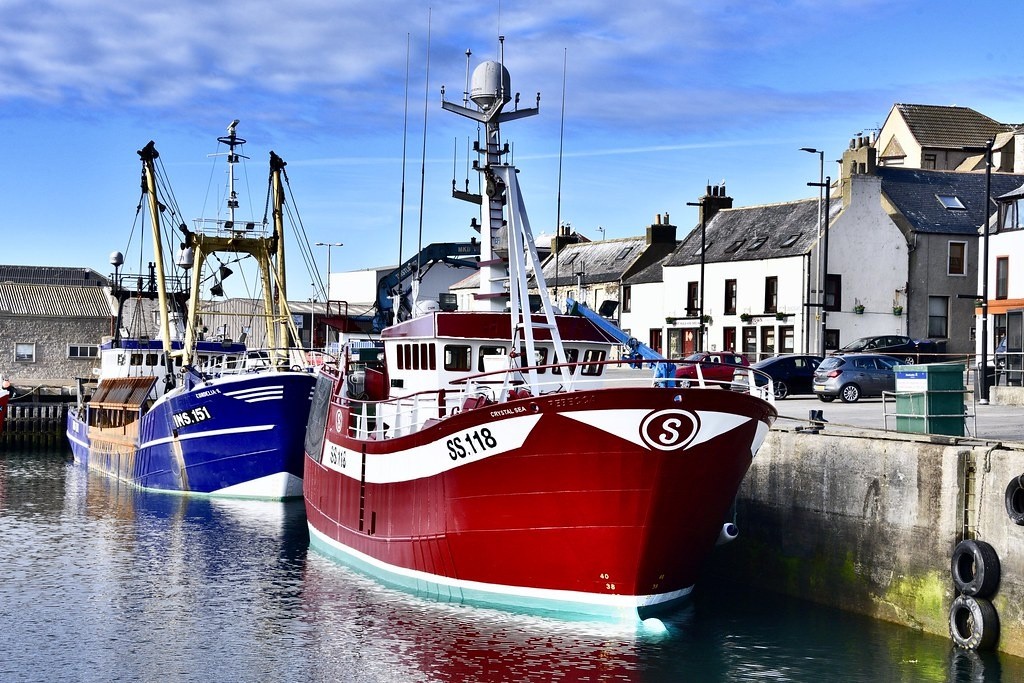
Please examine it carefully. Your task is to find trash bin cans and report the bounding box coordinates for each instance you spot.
[971,366,1002,401]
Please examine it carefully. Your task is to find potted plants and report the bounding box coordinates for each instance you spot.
[974,300,982,307]
[893,306,903,316]
[701,315,710,323]
[740,314,750,322]
[666,317,674,324]
[776,312,783,320]
[855,305,865,314]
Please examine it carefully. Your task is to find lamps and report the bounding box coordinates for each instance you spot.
[224,221,234,229]
[119,327,130,339]
[238,333,247,343]
[599,300,620,318]
[529,295,542,314]
[246,224,255,230]
[221,339,232,349]
[438,292,458,312]
[139,335,150,345]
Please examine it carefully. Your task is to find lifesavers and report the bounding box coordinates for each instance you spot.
[1004,475,1024,527]
[949,595,1000,651]
[951,539,1001,598]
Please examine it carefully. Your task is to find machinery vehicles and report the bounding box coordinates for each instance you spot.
[564,296,678,389]
[371,241,544,353]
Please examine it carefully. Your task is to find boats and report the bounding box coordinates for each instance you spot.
[299,4,780,627]
[64,122,338,502]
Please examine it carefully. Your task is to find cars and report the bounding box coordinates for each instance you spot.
[829,335,917,365]
[675,350,751,390]
[811,351,912,403]
[730,354,827,401]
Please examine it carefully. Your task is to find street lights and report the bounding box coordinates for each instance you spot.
[313,241,345,347]
[804,177,832,358]
[961,140,989,405]
[797,145,820,356]
[685,200,708,354]
[310,283,315,351]
